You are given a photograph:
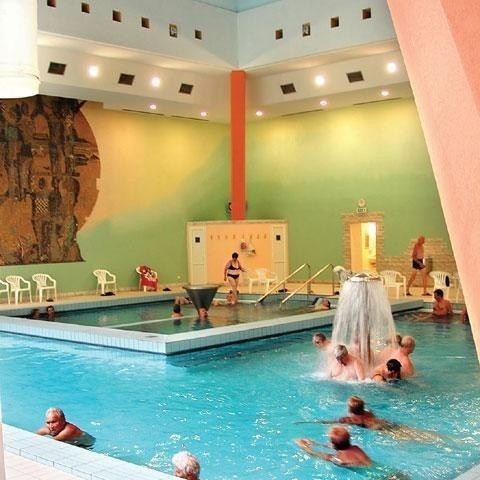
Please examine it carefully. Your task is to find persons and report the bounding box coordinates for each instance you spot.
[30,308,40,318]
[294,394,460,451]
[33,407,96,449]
[292,425,410,480]
[224,252,248,305]
[199,308,208,322]
[175,289,239,305]
[171,304,183,318]
[405,235,433,296]
[310,298,330,311]
[416,288,451,321]
[311,326,417,392]
[172,450,201,479]
[460,303,468,321]
[46,305,56,320]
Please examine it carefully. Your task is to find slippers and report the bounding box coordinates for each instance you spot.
[421,293,432,295]
[405,293,411,296]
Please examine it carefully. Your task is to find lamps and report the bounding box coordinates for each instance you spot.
[1,0,40,98]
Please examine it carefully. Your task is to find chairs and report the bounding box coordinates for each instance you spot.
[429,271,464,301]
[0,274,57,304]
[379,270,407,300]
[93,269,117,296]
[135,267,158,292]
[246,268,278,292]
